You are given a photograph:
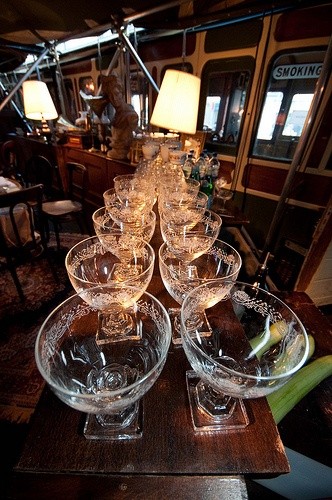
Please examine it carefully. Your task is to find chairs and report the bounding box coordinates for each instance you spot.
[0,140,93,302]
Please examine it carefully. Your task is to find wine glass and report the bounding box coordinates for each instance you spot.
[102,187,157,259]
[158,234,242,344]
[92,204,157,287]
[158,188,208,259]
[65,233,155,345]
[35,286,172,441]
[217,181,234,216]
[181,280,309,432]
[160,205,223,287]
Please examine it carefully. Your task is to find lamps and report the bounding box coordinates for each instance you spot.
[149,69,201,134]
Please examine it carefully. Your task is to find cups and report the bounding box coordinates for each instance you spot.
[102,132,199,225]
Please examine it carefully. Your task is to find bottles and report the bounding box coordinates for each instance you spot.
[85,113,112,156]
[183,149,220,209]
[135,110,148,134]
[228,250,284,353]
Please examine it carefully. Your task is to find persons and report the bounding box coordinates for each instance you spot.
[100,80,138,152]
